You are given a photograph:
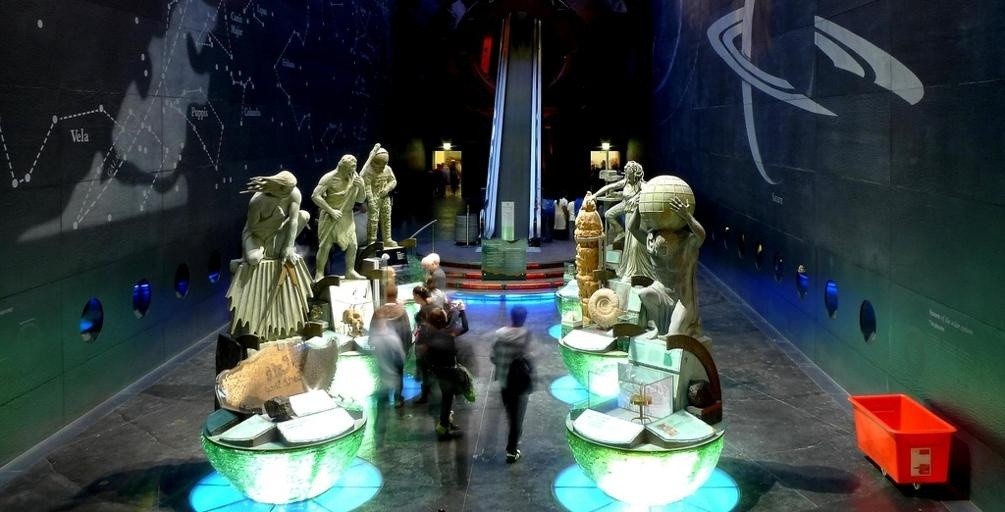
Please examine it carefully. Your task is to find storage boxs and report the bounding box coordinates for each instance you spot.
[848,393,957,489]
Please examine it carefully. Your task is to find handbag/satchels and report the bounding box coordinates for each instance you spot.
[444,361,472,395]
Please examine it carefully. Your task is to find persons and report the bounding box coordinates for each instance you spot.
[425,299,475,438]
[366,284,404,408]
[420,253,445,289]
[362,145,400,249]
[541,190,584,244]
[240,168,311,266]
[584,159,645,245]
[626,196,706,337]
[488,304,534,464]
[435,160,460,199]
[410,286,433,407]
[310,153,368,282]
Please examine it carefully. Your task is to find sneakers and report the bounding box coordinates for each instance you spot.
[506,448,521,463]
[436,423,462,435]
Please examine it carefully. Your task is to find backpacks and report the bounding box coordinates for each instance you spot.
[506,357,534,397]
